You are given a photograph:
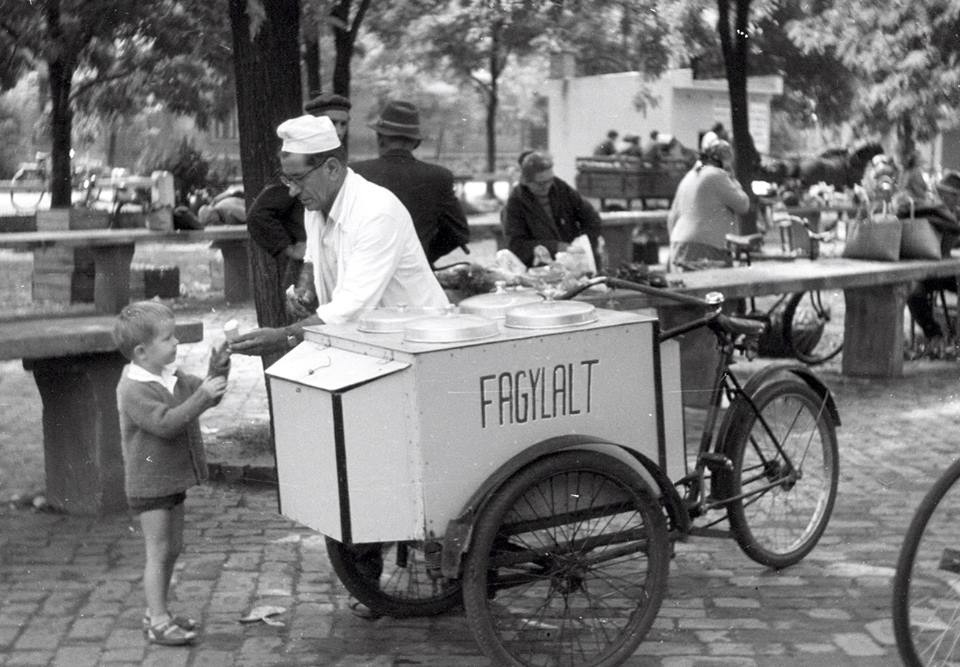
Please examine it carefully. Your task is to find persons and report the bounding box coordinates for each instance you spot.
[247,92,465,321]
[669,139,750,276]
[594,121,728,211]
[889,168,949,337]
[110,301,232,644]
[226,115,450,355]
[500,149,605,273]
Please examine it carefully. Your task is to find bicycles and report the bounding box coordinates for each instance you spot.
[9,150,90,211]
[671,214,843,364]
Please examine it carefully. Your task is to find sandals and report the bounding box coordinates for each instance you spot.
[141,612,196,634]
[144,622,196,646]
[347,592,377,618]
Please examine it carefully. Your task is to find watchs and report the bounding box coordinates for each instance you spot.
[285,326,298,350]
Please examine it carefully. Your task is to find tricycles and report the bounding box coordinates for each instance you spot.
[261,272,844,667]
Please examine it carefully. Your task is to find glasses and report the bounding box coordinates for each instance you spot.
[279,156,334,188]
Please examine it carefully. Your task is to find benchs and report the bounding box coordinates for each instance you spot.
[564,253,960,411]
[577,155,693,212]
[0,316,202,514]
[0,202,864,315]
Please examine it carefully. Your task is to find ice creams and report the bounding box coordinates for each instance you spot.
[223,320,238,344]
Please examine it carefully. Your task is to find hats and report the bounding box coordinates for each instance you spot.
[305,93,352,122]
[276,114,342,153]
[366,100,426,141]
